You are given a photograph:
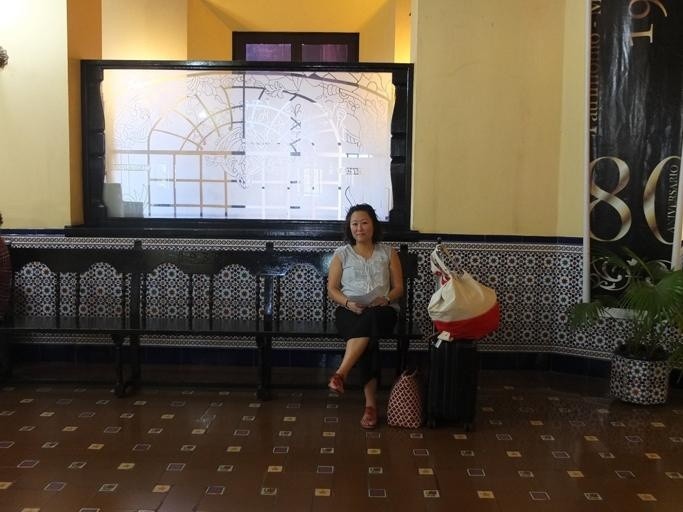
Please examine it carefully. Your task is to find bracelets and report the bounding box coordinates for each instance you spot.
[385,296,390,305]
[345,300,349,310]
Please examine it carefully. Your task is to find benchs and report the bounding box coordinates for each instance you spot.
[4,238,418,403]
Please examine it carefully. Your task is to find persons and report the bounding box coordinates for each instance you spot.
[328,205,404,428]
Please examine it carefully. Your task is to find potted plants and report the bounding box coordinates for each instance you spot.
[564,243,683,406]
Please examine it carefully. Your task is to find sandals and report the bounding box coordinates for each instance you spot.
[359,406,378,429]
[326,374,347,395]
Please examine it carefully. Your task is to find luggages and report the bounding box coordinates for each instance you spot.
[425,247,477,432]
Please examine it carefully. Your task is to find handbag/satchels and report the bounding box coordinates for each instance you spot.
[426,244,500,341]
[386,364,424,429]
